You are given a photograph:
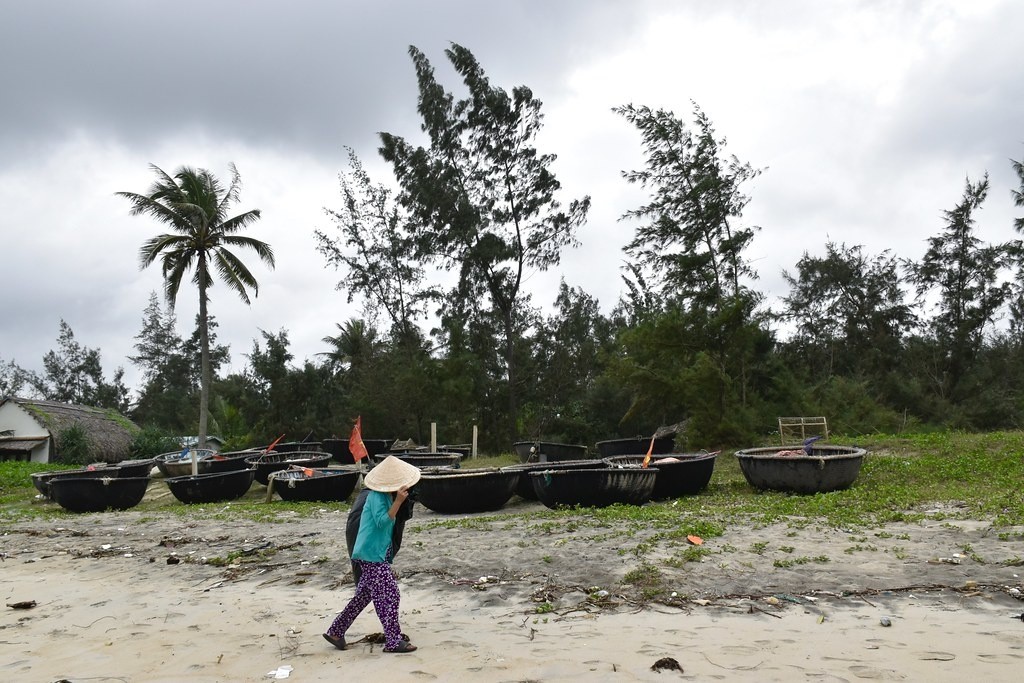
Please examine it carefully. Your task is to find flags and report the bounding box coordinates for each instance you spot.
[643,440,654,468]
[349,419,368,461]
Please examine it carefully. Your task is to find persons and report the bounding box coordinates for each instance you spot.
[322,455,421,652]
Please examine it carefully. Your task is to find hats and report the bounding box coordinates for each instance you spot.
[364,456,423,492]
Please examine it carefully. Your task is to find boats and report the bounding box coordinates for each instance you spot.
[734,444,868,495]
[501,459,604,502]
[602,451,718,502]
[48,476,152,513]
[323,438,394,465]
[245,450,333,486]
[529,468,662,512]
[374,443,525,515]
[30,466,122,501]
[165,468,258,506]
[595,437,675,459]
[514,440,587,464]
[269,467,361,505]
[92,441,323,478]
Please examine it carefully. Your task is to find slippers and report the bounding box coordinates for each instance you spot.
[323,633,348,650]
[383,640,417,652]
